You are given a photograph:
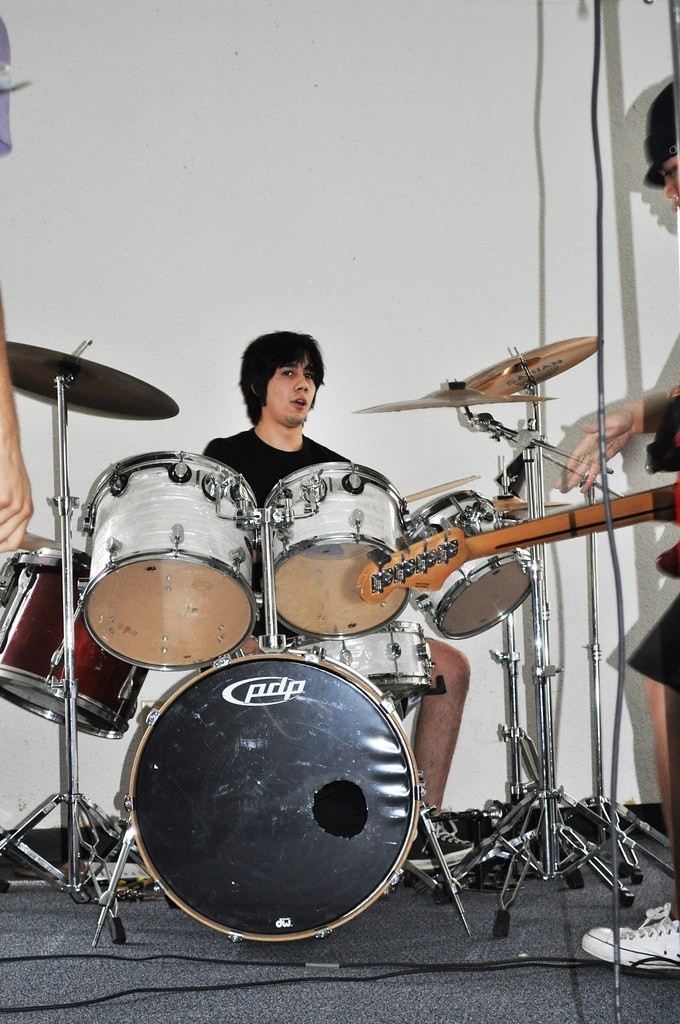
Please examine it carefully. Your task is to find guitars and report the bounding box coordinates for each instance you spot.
[354,382,680,605]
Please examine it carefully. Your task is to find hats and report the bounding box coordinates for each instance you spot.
[643,81,677,188]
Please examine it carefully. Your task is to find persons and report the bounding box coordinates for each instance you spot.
[203,331,472,859]
[555,82,680,969]
[0,293,34,552]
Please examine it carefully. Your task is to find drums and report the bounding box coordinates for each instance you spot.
[0,548,150,742]
[404,489,533,643]
[82,450,259,672]
[266,461,413,640]
[127,650,421,944]
[290,616,434,697]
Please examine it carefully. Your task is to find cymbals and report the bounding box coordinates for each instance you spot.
[350,387,558,414]
[464,335,604,397]
[493,493,571,519]
[5,338,184,422]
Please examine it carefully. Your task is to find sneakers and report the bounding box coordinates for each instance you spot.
[582,901,680,971]
[406,820,475,869]
